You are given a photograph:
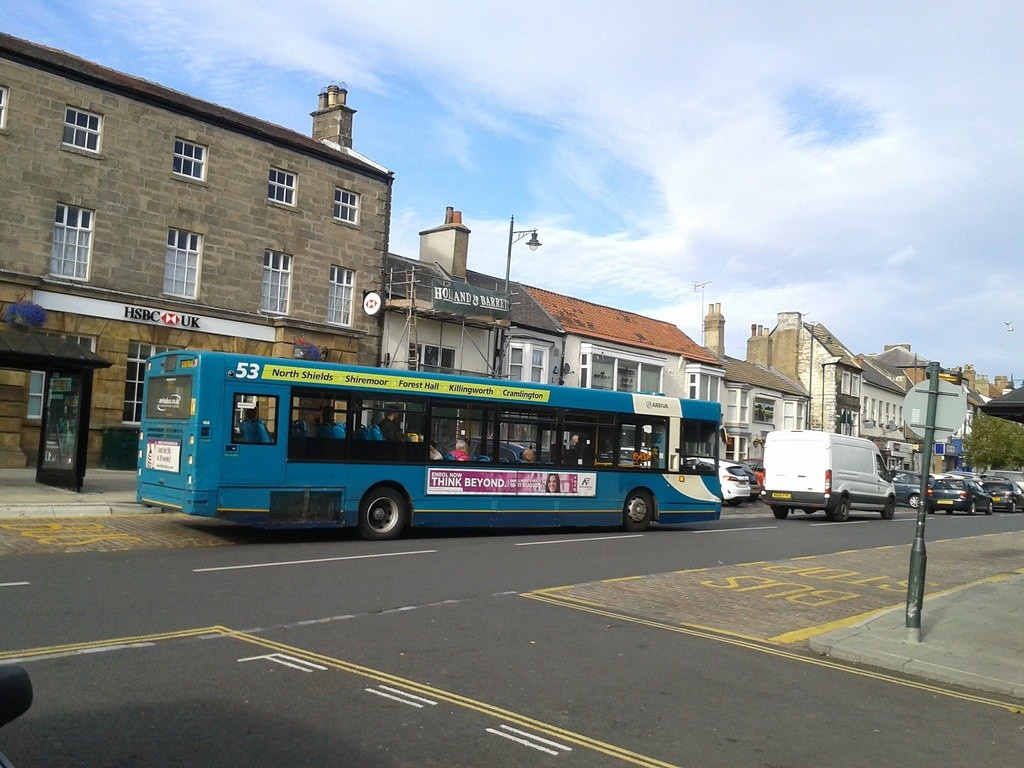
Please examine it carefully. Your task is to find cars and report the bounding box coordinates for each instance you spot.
[927,469,1024,514]
[929,478,995,516]
[889,468,934,508]
[598,447,765,504]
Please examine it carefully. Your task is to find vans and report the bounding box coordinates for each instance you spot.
[762,430,898,521]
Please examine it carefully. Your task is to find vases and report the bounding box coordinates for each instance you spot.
[295,348,304,359]
[11,313,31,330]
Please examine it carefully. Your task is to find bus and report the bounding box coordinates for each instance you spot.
[135,348,731,542]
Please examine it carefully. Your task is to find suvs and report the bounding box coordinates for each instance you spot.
[439,434,554,464]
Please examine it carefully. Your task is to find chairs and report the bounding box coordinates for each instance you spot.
[238,415,388,444]
[430,448,553,464]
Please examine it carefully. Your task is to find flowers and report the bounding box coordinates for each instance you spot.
[292,339,320,360]
[5,300,47,329]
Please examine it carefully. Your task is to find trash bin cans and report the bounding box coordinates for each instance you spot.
[101,425,140,471]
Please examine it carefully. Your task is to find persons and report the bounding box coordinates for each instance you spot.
[379,406,580,464]
[57,407,71,436]
[544,474,560,494]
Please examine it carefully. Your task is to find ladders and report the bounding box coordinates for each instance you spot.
[405,267,418,372]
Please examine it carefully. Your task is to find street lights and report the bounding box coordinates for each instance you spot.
[497,215,545,380]
